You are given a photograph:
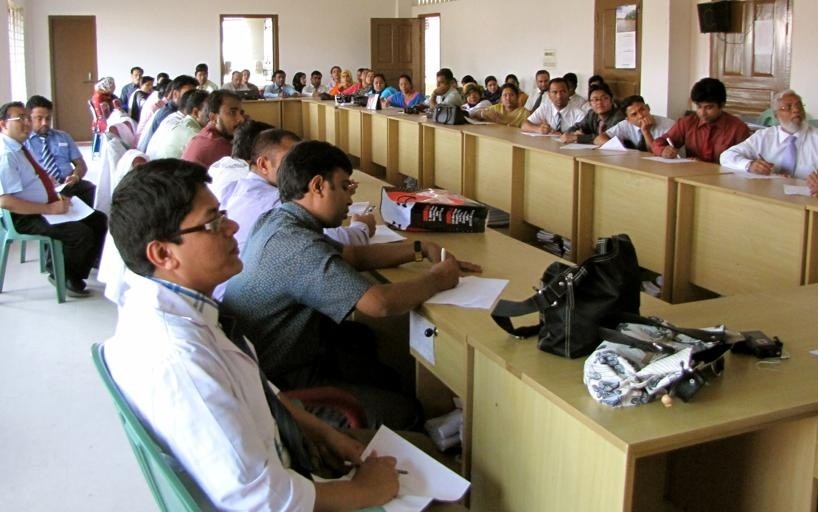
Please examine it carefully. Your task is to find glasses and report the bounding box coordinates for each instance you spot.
[5,115,33,122]
[175,210,228,237]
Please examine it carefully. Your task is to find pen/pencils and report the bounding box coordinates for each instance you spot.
[440,247,447,261]
[666,138,681,158]
[57,192,63,200]
[592,146,601,150]
[343,461,408,475]
[759,153,773,170]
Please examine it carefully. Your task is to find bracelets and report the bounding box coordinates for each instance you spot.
[71,174,80,183]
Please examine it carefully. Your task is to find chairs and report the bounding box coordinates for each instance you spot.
[90,341,205,511]
[0,205,66,304]
[87,98,104,160]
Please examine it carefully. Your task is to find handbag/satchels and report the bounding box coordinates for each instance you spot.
[432,103,463,124]
[582,313,733,409]
[380,186,489,233]
[492,232,643,359]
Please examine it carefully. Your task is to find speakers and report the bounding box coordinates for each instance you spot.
[697,3,743,33]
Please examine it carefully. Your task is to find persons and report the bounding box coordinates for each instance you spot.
[0,101,108,297]
[22,95,97,209]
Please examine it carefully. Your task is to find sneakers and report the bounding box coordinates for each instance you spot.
[45,246,91,298]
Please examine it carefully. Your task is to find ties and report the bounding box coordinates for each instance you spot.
[778,135,798,177]
[39,135,62,183]
[21,146,59,202]
[532,92,543,113]
[555,113,563,132]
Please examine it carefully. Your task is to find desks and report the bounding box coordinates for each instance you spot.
[237,93,816,511]
